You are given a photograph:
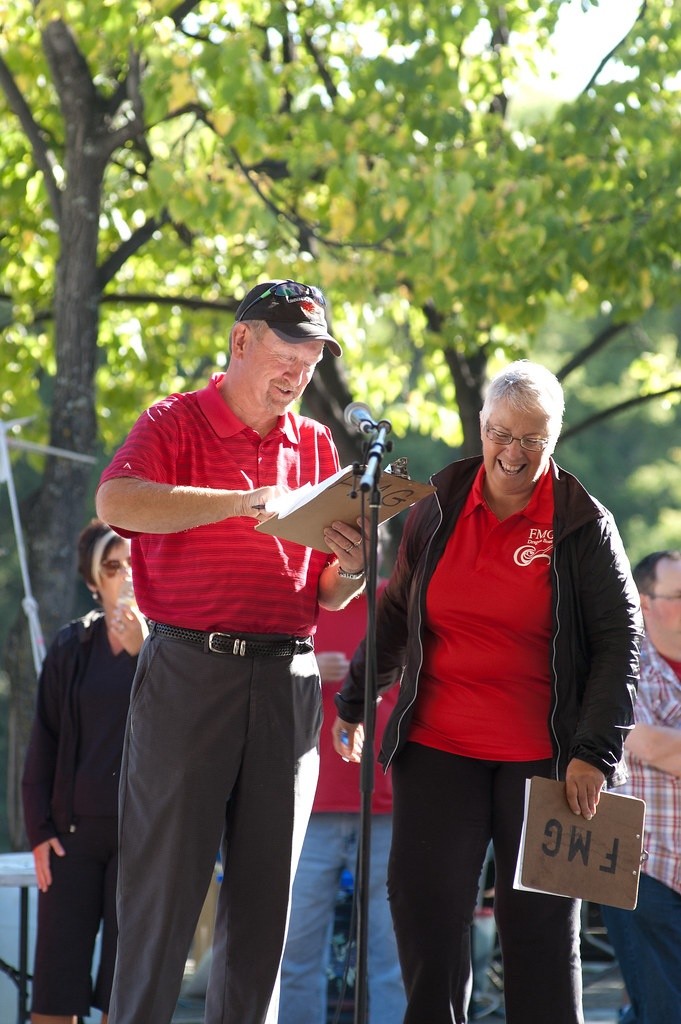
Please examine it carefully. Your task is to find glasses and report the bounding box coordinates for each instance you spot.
[486,423,548,452]
[238,282,327,322]
[99,556,131,577]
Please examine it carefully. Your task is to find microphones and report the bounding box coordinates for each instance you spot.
[344,402,374,434]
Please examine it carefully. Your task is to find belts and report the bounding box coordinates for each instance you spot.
[147,618,316,657]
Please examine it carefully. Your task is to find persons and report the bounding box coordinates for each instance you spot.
[23,520,149,1021]
[600,552,681,1024]
[97,281,375,1024]
[277,576,406,1022]
[333,359,643,1024]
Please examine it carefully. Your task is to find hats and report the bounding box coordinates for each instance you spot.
[235,280,342,361]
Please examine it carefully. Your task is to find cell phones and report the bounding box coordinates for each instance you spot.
[116,578,138,604]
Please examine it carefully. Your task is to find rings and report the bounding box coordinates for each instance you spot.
[119,622,126,633]
[345,544,355,552]
[354,537,363,547]
